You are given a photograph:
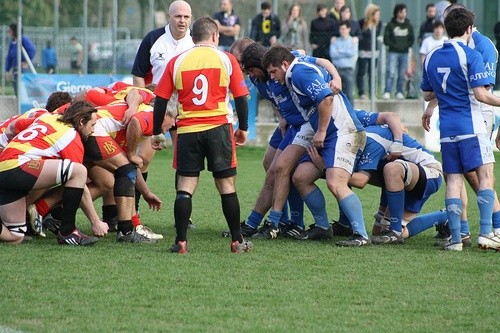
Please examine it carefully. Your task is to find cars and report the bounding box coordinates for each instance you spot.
[87,38,143,72]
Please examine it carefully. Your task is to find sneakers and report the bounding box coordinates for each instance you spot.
[330,219,353,235]
[103,215,118,232]
[435,208,452,241]
[43,213,62,235]
[135,225,164,240]
[222,221,257,238]
[371,229,404,244]
[278,220,306,238]
[460,231,472,247]
[116,227,158,244]
[57,228,98,245]
[231,234,252,253]
[166,240,187,254]
[250,219,281,239]
[476,231,500,251]
[434,237,463,251]
[293,224,332,240]
[26,203,46,237]
[334,231,372,248]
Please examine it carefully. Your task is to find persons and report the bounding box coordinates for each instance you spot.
[0,0,500,253]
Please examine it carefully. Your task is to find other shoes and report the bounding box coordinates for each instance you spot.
[396,91,404,100]
[383,91,390,99]
[360,93,366,98]
